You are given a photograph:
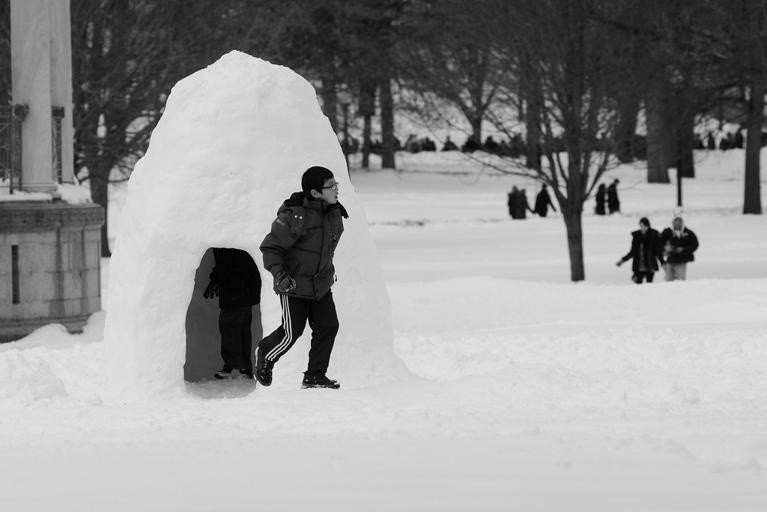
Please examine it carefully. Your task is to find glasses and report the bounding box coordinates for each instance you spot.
[316,182,339,191]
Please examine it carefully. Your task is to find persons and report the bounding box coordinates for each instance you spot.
[203,248,261,379]
[616,217,698,283]
[595,179,619,214]
[254,166,348,389]
[508,183,556,219]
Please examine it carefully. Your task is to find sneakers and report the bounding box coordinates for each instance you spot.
[239,366,254,379]
[252,347,274,387]
[298,369,342,390]
[213,363,233,381]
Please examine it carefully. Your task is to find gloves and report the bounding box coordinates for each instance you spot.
[276,273,296,295]
